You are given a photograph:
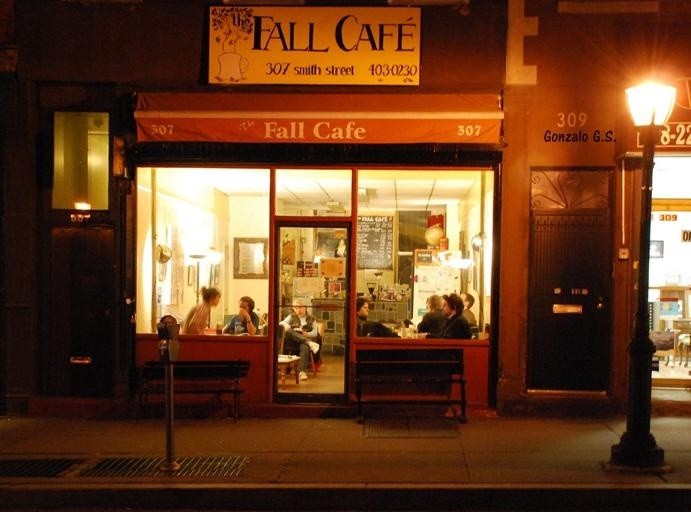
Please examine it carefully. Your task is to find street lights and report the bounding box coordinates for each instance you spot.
[606,66,679,474]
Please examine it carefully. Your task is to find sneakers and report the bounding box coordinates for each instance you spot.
[300,371,308,379]
[311,341,320,354]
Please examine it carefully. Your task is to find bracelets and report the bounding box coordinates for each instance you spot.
[245,316,252,323]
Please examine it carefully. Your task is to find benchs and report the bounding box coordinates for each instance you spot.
[355,348,469,424]
[137,359,251,423]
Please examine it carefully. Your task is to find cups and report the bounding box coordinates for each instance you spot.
[235,321,242,336]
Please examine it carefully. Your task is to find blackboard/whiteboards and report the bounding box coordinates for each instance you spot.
[356,216,393,269]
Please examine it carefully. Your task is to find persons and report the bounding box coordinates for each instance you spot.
[418,292,478,338]
[222,296,260,335]
[182,287,222,335]
[280,297,321,380]
[357,297,371,336]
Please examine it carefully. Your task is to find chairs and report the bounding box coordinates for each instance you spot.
[262,318,326,390]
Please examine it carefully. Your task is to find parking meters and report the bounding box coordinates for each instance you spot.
[155,314,182,473]
